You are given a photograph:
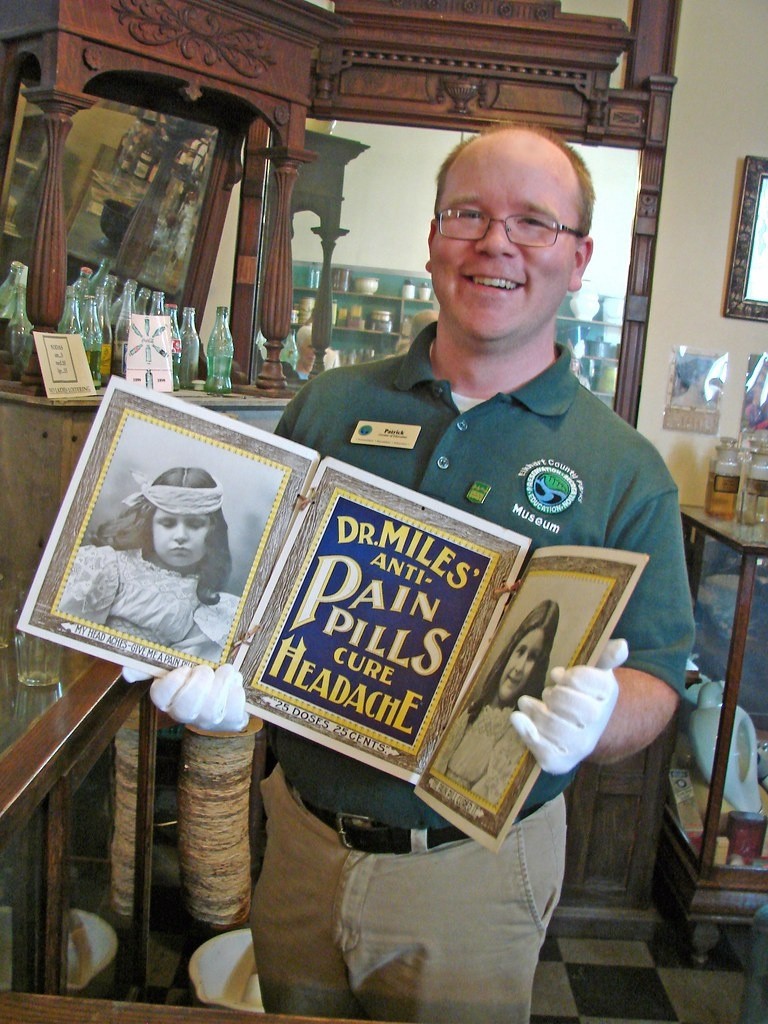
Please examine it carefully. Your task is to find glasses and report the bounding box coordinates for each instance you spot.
[435,207,584,247]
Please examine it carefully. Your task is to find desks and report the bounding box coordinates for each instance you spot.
[66,143,180,296]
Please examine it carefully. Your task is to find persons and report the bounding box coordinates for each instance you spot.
[295,327,315,381]
[388,308,438,355]
[433,599,560,809]
[57,467,245,665]
[745,371,768,430]
[124,119,695,1024]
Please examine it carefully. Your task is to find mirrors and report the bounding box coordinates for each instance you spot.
[722,157,768,322]
[0,77,219,296]
[248,116,643,411]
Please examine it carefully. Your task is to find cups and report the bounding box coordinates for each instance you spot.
[338,305,347,319]
[351,304,362,320]
[331,267,350,292]
[370,320,393,334]
[0,562,17,646]
[729,810,768,864]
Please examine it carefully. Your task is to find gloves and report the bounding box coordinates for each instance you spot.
[509,637,630,775]
[121,663,250,732]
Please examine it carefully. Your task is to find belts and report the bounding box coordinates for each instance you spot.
[297,795,546,857]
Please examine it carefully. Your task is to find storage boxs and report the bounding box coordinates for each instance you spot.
[667,768,704,834]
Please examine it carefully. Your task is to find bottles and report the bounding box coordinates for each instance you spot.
[705,437,742,520]
[278,328,298,369]
[308,262,320,288]
[738,440,768,526]
[418,281,432,300]
[164,304,181,391]
[149,291,165,316]
[403,278,415,299]
[58,286,112,390]
[8,568,61,686]
[204,306,233,393]
[0,261,28,318]
[180,307,199,389]
[569,277,601,320]
[112,282,136,379]
[71,259,151,325]
[291,297,316,324]
[7,285,34,373]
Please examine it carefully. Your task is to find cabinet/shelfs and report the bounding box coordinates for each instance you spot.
[0,639,161,1003]
[654,505,768,972]
[292,288,623,398]
[0,0,354,739]
[232,0,680,940]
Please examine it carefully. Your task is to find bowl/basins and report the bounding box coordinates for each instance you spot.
[187,928,265,1012]
[589,336,613,359]
[355,277,380,295]
[62,908,118,998]
[0,905,12,992]
